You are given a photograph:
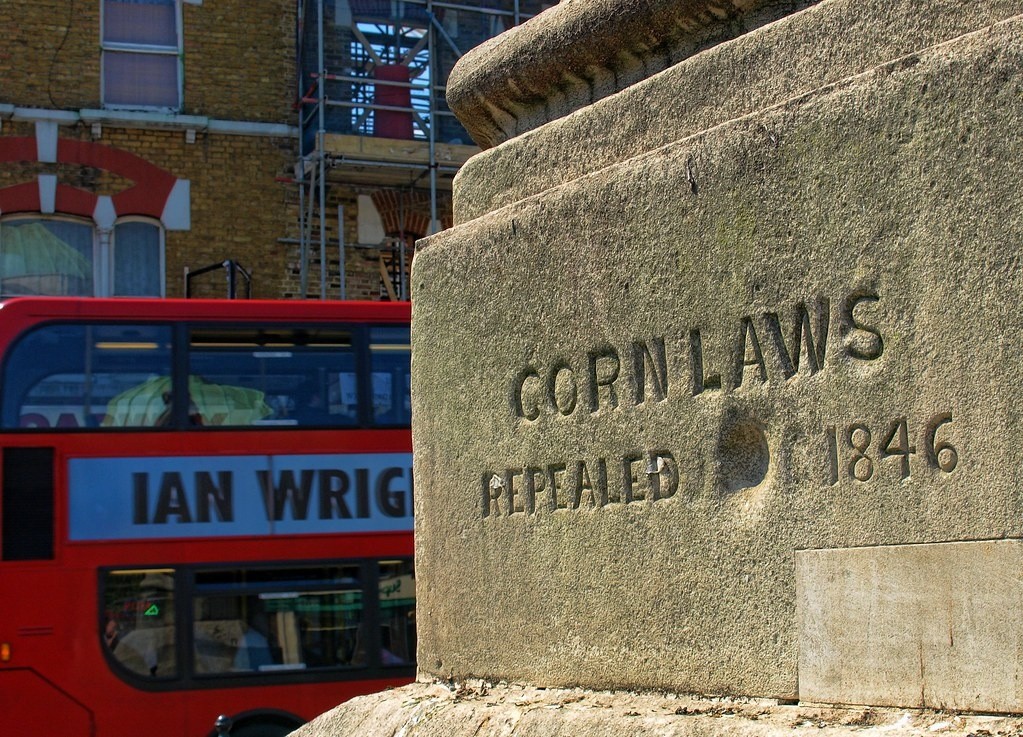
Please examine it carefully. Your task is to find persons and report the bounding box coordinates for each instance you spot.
[105,620,120,648]
[233,608,273,670]
[290,383,323,418]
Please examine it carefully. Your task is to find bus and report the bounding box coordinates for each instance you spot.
[1,287,422,737]
